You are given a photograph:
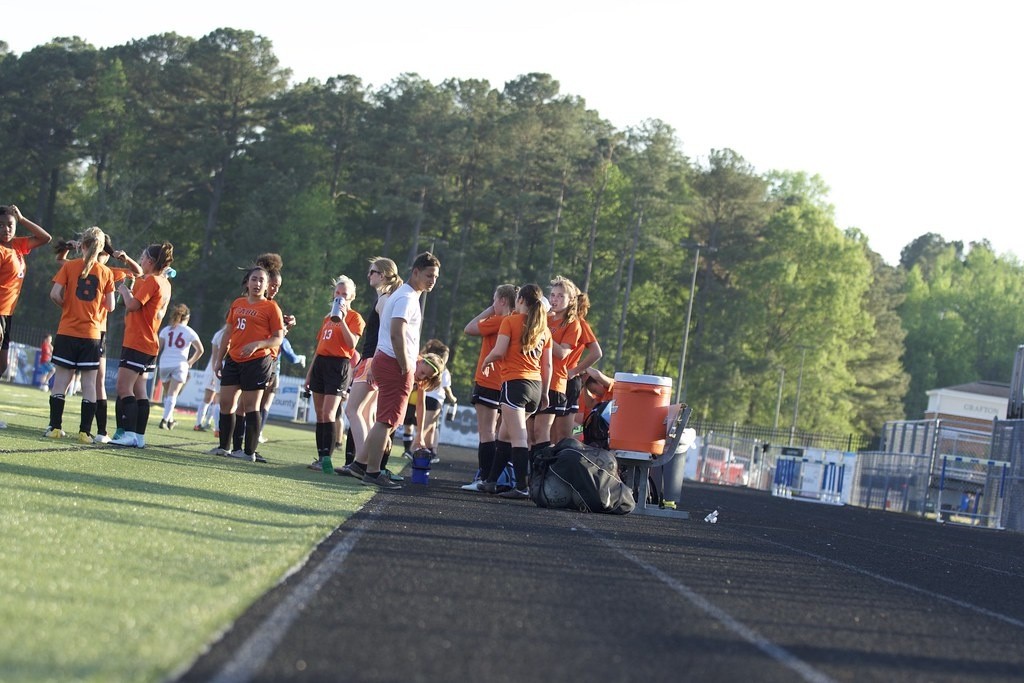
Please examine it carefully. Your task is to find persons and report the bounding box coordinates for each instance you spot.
[334,258,444,482]
[111,242,173,449]
[402,339,457,463]
[214,267,284,461]
[45,227,115,444]
[68,370,81,397]
[345,252,441,488]
[244,324,306,443]
[0,205,53,429]
[305,275,366,475]
[158,303,205,430]
[40,334,56,393]
[462,276,614,500]
[231,254,297,463]
[194,311,225,437]
[55,234,145,444]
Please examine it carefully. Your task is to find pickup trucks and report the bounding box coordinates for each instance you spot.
[697,443,745,485]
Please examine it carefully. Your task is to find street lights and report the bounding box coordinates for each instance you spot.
[673,242,717,405]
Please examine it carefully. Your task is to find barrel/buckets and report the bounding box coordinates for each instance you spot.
[608,371,674,456]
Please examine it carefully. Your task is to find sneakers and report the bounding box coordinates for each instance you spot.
[195,424,208,432]
[97,435,111,443]
[136,434,145,448]
[322,456,334,474]
[336,461,404,481]
[204,448,232,456]
[460,471,530,499]
[309,459,323,471]
[43,428,63,439]
[80,433,94,444]
[169,421,179,429]
[160,418,168,429]
[335,442,344,451]
[362,472,402,489]
[403,447,440,463]
[112,432,138,446]
[255,453,266,463]
[227,450,256,461]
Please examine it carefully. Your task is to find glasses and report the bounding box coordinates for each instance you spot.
[370,270,380,275]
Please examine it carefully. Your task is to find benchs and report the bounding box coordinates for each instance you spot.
[610,404,695,520]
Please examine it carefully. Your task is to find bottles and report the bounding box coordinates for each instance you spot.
[330,296,345,323]
[412,449,432,484]
[116,273,136,304]
[167,268,177,278]
